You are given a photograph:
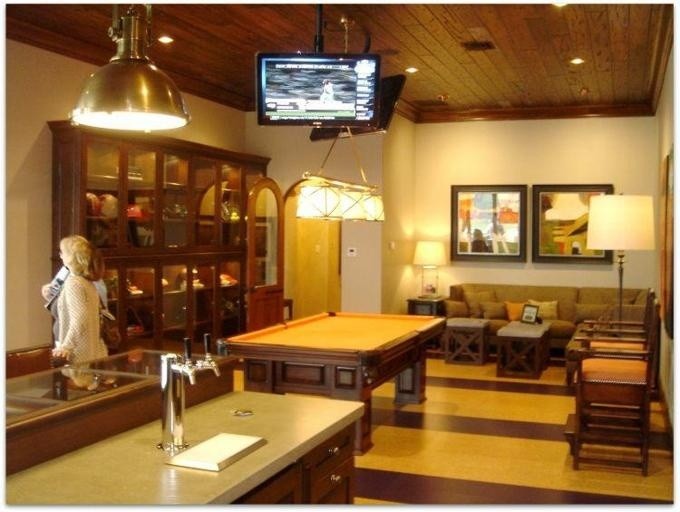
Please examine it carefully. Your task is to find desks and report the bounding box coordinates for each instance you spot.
[6,348,236,478]
[225,310,447,456]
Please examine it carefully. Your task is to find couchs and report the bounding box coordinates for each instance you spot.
[442,282,662,389]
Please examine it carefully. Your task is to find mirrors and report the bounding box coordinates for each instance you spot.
[245,177,285,333]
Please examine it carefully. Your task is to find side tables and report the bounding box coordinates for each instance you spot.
[407,296,446,351]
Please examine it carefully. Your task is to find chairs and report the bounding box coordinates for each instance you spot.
[565,288,662,478]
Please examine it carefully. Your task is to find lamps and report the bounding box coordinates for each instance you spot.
[291,15,387,224]
[66,4,191,133]
[584,193,658,335]
[412,241,447,299]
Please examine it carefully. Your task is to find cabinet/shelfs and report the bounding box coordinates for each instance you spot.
[302,407,357,503]
[47,120,272,369]
[239,445,302,506]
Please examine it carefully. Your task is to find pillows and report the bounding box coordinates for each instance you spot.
[446,291,613,322]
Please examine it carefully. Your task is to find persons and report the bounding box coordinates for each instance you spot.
[320,79,335,105]
[50,235,108,367]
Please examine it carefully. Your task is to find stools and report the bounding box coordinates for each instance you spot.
[496,327,543,380]
[444,317,491,367]
[505,320,552,372]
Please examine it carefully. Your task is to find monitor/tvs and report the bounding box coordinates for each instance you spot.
[255,51,378,130]
[310,74,407,140]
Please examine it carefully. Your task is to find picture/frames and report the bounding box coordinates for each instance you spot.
[448,184,527,261]
[531,183,615,264]
[521,304,543,324]
